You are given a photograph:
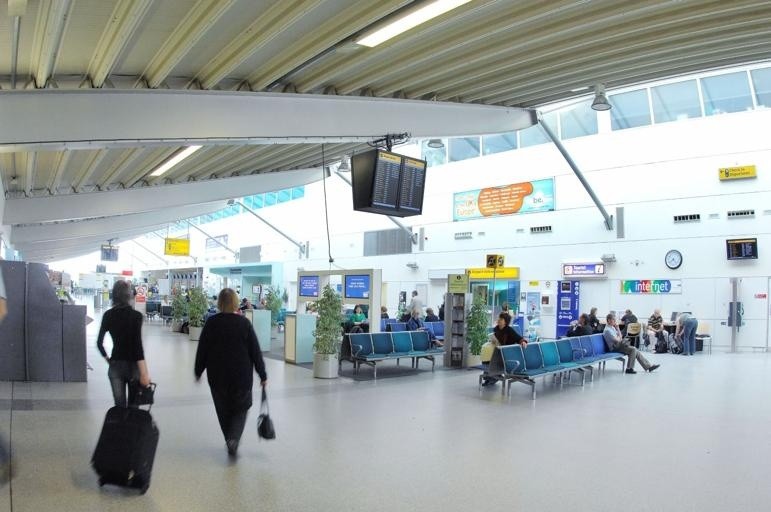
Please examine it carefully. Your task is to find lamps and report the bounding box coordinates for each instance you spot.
[355,1,474,47]
[337,157,350,173]
[428,140,444,148]
[591,93,612,111]
[151,144,205,179]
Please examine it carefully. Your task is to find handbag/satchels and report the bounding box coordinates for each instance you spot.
[656,341,667,352]
[258,414,275,439]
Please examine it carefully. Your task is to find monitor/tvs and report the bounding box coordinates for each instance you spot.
[345,274,370,299]
[252,285,262,294]
[299,276,319,297]
[725,238,758,260]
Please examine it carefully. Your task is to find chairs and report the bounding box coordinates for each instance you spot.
[339,312,447,380]
[474,332,632,400]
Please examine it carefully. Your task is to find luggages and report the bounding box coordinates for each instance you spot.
[481,339,495,379]
[92,383,158,495]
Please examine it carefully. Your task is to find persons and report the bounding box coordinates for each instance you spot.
[674,312,699,355]
[349,304,367,333]
[602,314,660,374]
[193,287,268,456]
[1,266,9,322]
[620,309,638,338]
[380,290,445,346]
[566,307,600,337]
[97,280,153,409]
[482,312,527,386]
[647,308,669,351]
[101,282,267,310]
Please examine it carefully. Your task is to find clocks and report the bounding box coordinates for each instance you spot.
[664,250,682,269]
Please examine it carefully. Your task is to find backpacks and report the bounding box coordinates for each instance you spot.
[669,333,683,353]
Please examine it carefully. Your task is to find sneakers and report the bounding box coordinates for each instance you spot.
[435,341,443,347]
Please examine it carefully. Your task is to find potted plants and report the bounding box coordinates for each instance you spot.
[465,293,490,367]
[187,285,210,342]
[312,285,346,380]
[171,289,187,333]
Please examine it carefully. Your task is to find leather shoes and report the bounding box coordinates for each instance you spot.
[626,369,636,373]
[649,364,660,371]
